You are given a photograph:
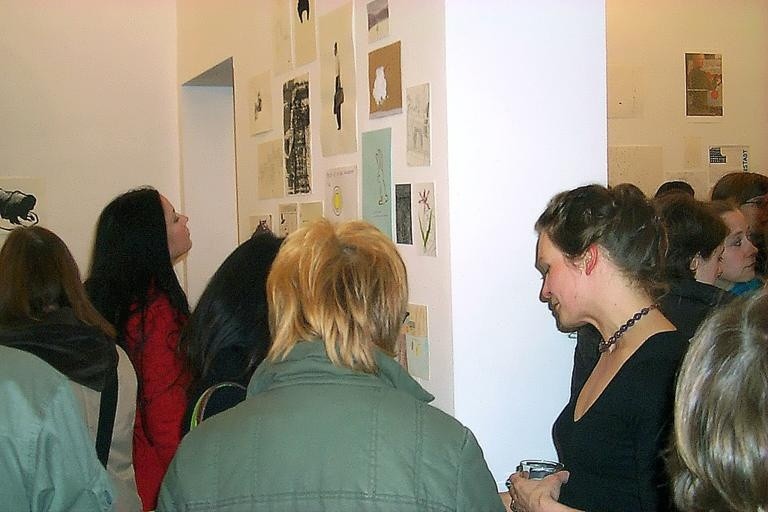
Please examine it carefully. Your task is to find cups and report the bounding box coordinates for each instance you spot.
[517,458,565,482]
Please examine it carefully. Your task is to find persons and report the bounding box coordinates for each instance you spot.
[153,214,510,510]
[602,170,766,343]
[2,225,146,510]
[332,42,344,132]
[504,184,690,511]
[178,228,292,446]
[659,280,767,512]
[80,186,196,510]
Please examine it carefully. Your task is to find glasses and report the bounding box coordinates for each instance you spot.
[743,200,767,209]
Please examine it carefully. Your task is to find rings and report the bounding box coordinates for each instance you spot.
[504,478,512,491]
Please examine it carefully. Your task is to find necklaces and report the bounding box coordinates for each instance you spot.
[596,301,663,355]
